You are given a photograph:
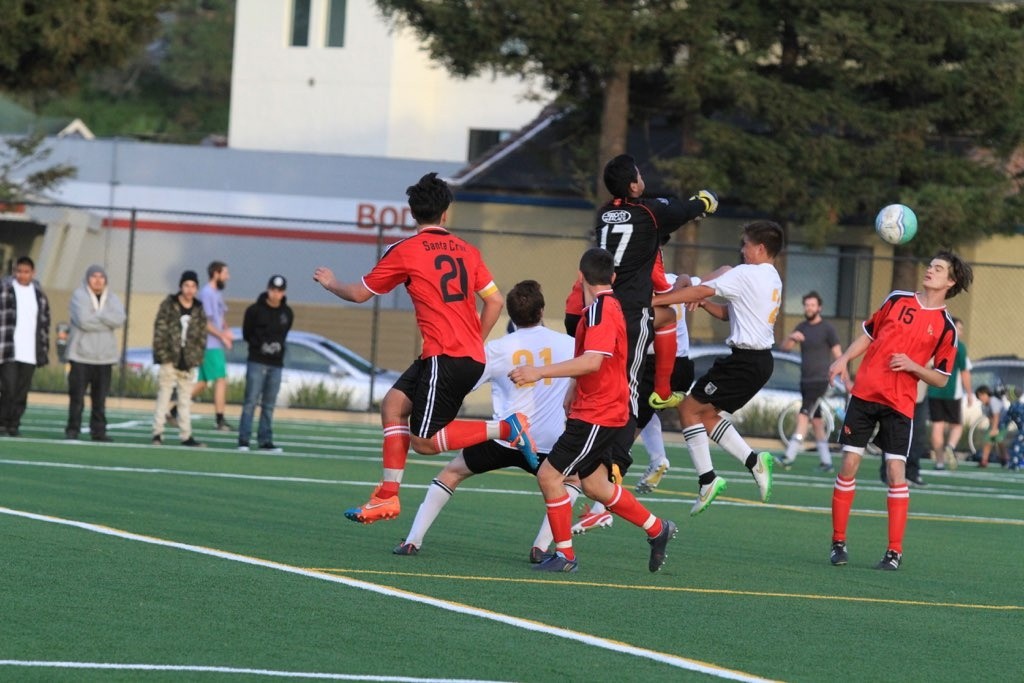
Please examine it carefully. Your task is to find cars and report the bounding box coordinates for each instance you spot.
[127,326,403,411]
[654,343,851,440]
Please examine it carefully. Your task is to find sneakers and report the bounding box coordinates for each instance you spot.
[533,552,578,573]
[635,458,669,495]
[502,411,539,470]
[571,504,613,536]
[690,476,726,515]
[392,543,419,556]
[881,550,904,569]
[647,521,679,573]
[752,453,775,504]
[830,542,848,565]
[647,391,685,411]
[816,463,835,473]
[773,455,792,472]
[344,488,400,525]
[530,547,555,564]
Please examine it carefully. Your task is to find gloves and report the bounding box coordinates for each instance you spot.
[697,189,718,213]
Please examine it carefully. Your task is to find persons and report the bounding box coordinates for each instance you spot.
[0,253,294,457]
[313,154,1024,575]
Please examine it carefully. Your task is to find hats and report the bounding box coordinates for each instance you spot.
[86,265,106,283]
[268,276,285,289]
[178,271,198,287]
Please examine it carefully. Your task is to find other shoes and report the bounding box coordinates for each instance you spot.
[66,411,283,453]
[1,427,18,437]
[907,472,928,487]
[945,445,958,471]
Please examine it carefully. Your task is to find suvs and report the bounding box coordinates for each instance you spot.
[924,355,1024,452]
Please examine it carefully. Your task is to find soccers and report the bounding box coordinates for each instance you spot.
[873,204,918,247]
